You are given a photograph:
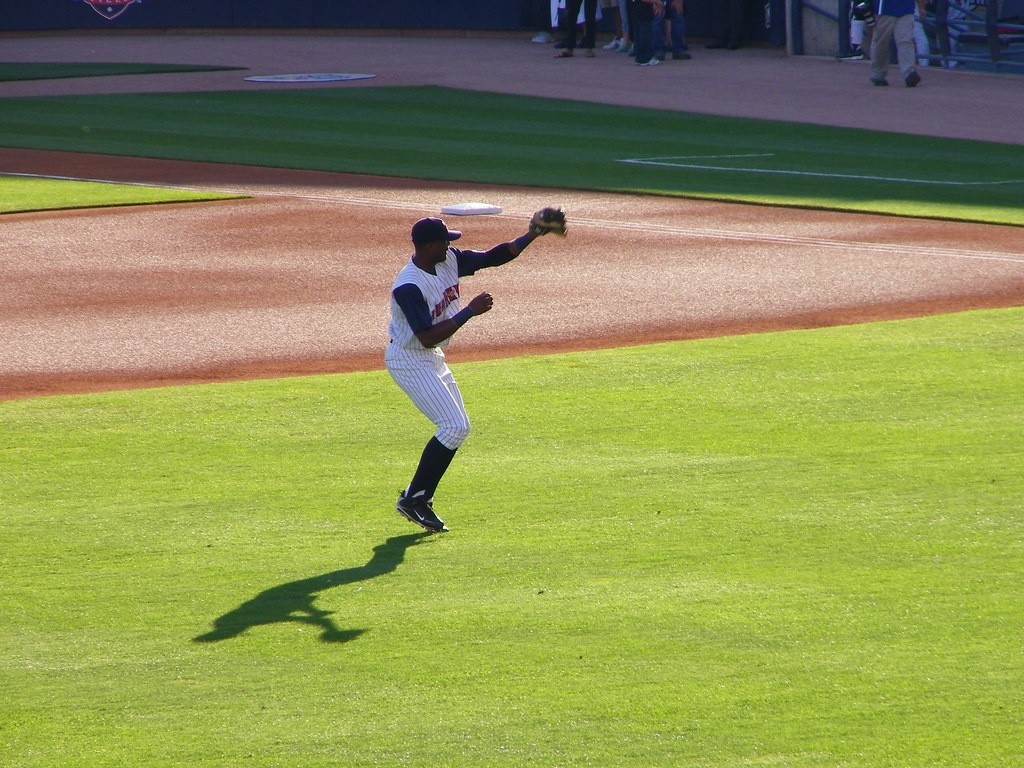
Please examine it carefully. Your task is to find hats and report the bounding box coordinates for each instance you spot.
[411,217,462,243]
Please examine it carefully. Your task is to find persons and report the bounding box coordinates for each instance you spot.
[705,0,743,50]
[531,0,692,66]
[385,208,567,531]
[835,0,930,86]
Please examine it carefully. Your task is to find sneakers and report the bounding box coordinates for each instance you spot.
[397,491,445,533]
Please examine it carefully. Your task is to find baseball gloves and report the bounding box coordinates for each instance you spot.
[527,206,568,238]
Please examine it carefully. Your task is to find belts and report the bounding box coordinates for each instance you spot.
[390,339,394,344]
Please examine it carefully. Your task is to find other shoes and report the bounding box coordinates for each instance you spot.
[553,50,574,58]
[627,43,638,56]
[671,50,692,59]
[635,57,660,66]
[905,70,922,88]
[532,31,557,43]
[869,77,889,87]
[584,49,595,58]
[617,42,632,52]
[835,48,864,60]
[602,40,620,50]
